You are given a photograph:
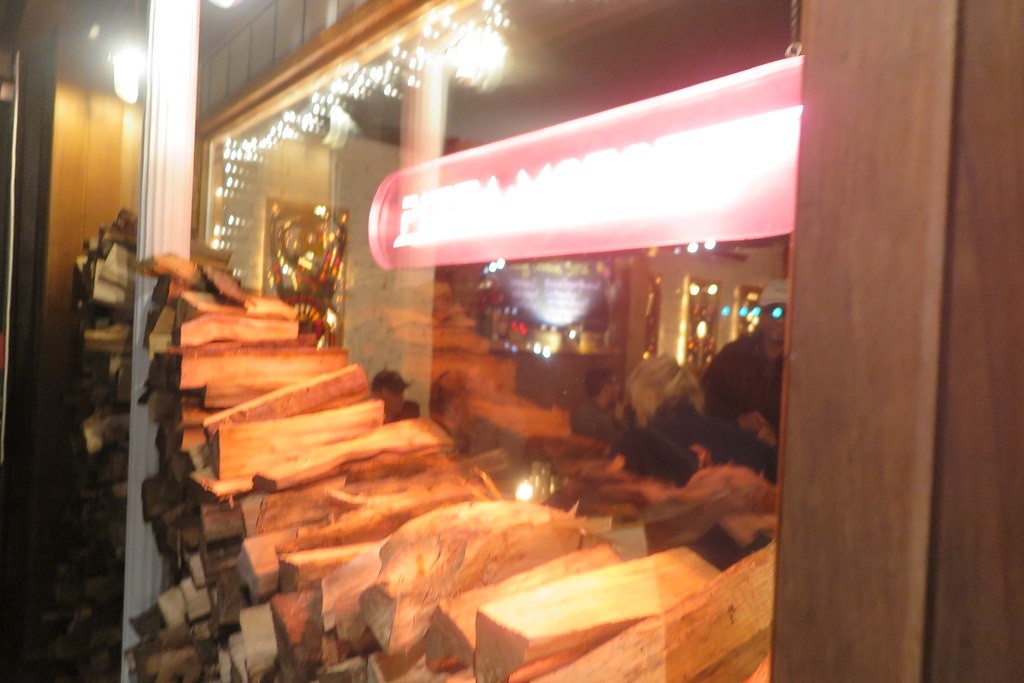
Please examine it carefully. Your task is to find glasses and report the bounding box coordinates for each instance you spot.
[610,379,620,386]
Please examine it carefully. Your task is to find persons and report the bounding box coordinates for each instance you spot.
[371,279,787,491]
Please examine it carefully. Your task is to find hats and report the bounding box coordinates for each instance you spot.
[757,279,788,308]
[373,371,410,392]
